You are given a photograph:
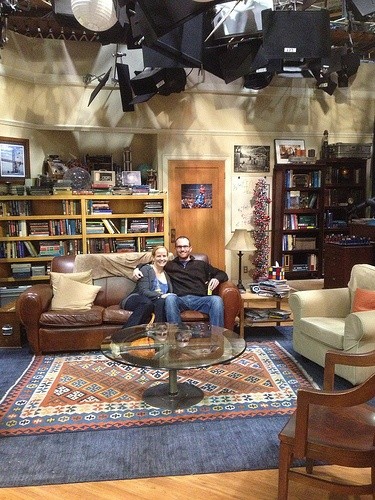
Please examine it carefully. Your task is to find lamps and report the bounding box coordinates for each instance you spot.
[224,228,257,287]
[129,53,360,104]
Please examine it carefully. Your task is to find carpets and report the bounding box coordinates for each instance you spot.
[0,326,375,488]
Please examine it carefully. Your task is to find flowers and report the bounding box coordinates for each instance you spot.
[252,177,271,282]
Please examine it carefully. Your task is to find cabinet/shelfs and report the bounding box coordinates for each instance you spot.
[0,192,169,282]
[271,161,375,288]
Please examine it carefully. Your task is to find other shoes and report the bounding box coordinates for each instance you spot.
[170,348,182,359]
[207,346,223,359]
[110,343,122,359]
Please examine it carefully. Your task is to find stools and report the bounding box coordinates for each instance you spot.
[0,300,25,348]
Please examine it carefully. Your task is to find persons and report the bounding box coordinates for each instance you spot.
[120,245,173,328]
[182,185,212,208]
[130,236,229,333]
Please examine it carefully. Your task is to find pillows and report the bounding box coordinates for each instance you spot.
[49,269,92,293]
[49,276,103,312]
[352,287,375,312]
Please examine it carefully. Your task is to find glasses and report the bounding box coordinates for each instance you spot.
[175,245,190,248]
[175,339,190,343]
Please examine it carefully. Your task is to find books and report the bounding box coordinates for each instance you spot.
[246,167,360,319]
[0,183,164,278]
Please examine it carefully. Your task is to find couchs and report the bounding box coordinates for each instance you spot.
[287,263,375,385]
[17,252,241,351]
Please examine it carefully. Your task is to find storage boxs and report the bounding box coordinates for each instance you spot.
[0,285,31,306]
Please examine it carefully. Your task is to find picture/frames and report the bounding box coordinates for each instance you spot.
[0,136,31,184]
[274,139,305,165]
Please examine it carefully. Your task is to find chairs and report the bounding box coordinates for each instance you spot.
[276,350,375,500]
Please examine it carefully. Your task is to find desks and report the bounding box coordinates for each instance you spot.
[237,283,302,335]
[101,321,246,409]
